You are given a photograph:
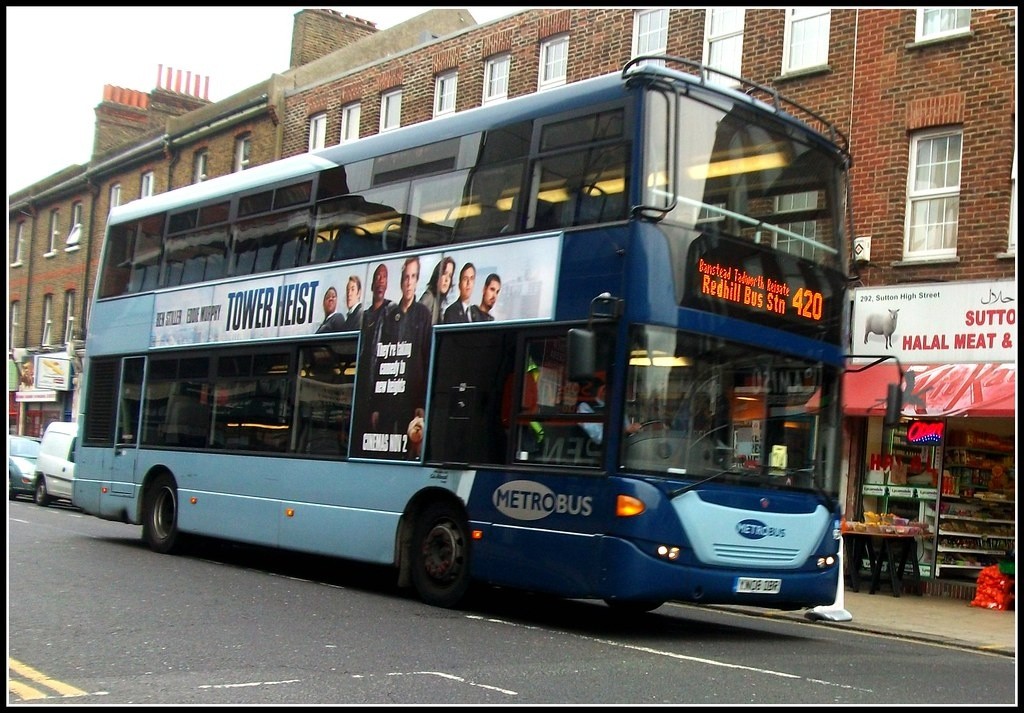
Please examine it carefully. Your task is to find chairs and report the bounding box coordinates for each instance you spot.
[127,188,629,294]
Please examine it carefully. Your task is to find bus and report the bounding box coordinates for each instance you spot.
[72,51,907,623]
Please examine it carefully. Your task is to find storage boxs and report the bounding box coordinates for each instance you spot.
[846,514,920,535]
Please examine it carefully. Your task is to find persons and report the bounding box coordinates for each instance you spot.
[316,256,501,462]
[577,382,641,451]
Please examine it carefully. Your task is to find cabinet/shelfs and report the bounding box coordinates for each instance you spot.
[880,421,1016,581]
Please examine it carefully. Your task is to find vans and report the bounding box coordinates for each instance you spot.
[33,420,77,506]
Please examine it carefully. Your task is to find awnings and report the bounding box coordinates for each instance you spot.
[806,363,1015,419]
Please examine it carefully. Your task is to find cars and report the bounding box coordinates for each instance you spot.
[8,435,43,498]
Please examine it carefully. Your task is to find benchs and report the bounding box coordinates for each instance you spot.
[167,400,212,448]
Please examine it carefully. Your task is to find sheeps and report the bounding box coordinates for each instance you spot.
[864,308,900,350]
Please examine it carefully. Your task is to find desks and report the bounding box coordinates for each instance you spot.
[844,532,934,597]
